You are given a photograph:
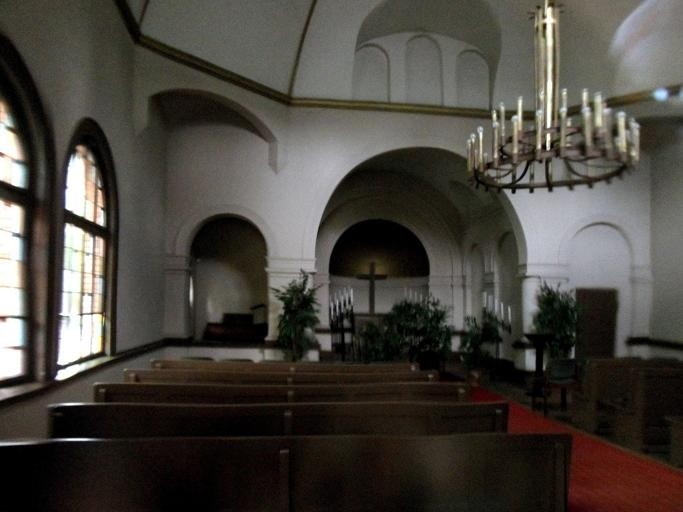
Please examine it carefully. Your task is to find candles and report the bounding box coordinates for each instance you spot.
[330,287,354,317]
[481,291,511,322]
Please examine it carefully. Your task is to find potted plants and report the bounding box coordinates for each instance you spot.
[362,300,500,379]
[533,284,589,385]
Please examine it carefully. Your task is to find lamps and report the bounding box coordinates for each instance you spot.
[466,1,641,193]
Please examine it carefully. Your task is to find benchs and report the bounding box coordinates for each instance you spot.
[0,358,574,512]
[573,356,682,465]
